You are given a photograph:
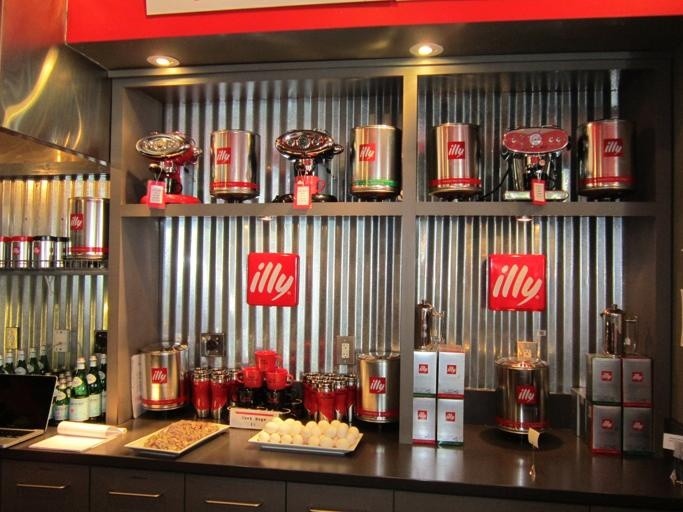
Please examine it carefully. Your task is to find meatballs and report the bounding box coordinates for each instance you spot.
[258,415,361,451]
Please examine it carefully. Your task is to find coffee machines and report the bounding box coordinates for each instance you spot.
[501,125,573,201]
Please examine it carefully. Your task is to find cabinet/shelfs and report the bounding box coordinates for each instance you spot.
[0,51,683,512]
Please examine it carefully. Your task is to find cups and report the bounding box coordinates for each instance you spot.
[235,367,264,389]
[299,175,326,195]
[252,350,282,371]
[266,368,294,390]
[156,177,182,196]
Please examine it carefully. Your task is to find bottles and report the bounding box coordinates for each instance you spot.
[600,303,640,355]
[1,345,108,428]
[302,372,357,424]
[192,368,242,421]
[1,234,69,271]
[417,298,444,349]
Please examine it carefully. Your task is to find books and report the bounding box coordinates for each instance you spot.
[28,420,128,453]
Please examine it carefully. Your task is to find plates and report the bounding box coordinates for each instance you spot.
[247,428,364,456]
[124,419,231,459]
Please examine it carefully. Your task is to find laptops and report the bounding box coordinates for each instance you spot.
[0,374,57,449]
